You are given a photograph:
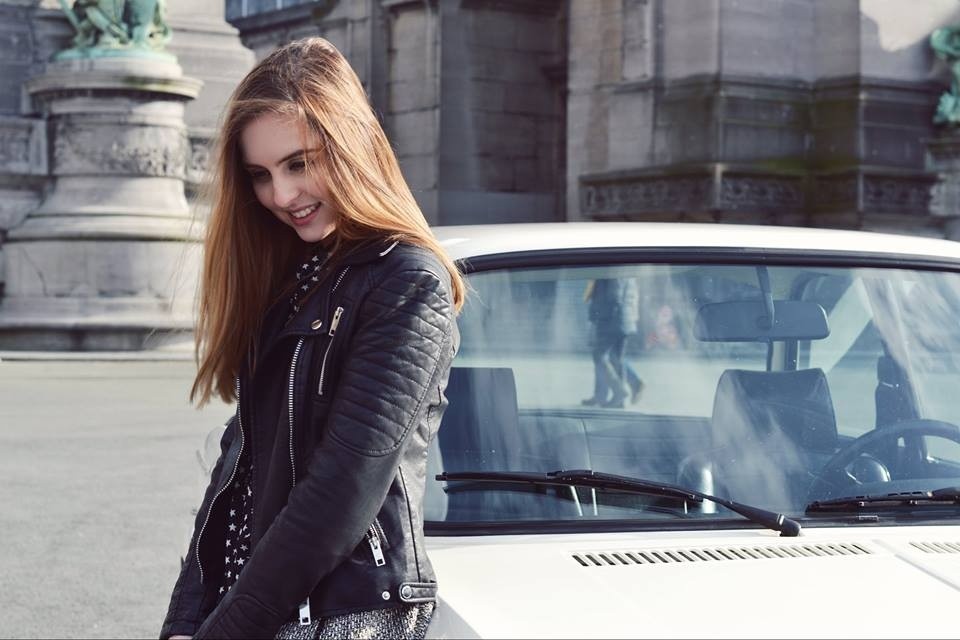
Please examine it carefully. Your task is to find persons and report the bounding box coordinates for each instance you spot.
[160,38,464,640]
[581,277,644,409]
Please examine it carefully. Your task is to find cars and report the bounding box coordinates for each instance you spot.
[419,223,960,640]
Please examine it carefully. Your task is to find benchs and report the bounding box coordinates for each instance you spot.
[514,410,714,487]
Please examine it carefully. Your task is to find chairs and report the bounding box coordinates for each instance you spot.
[438,365,519,474]
[715,367,838,499]
